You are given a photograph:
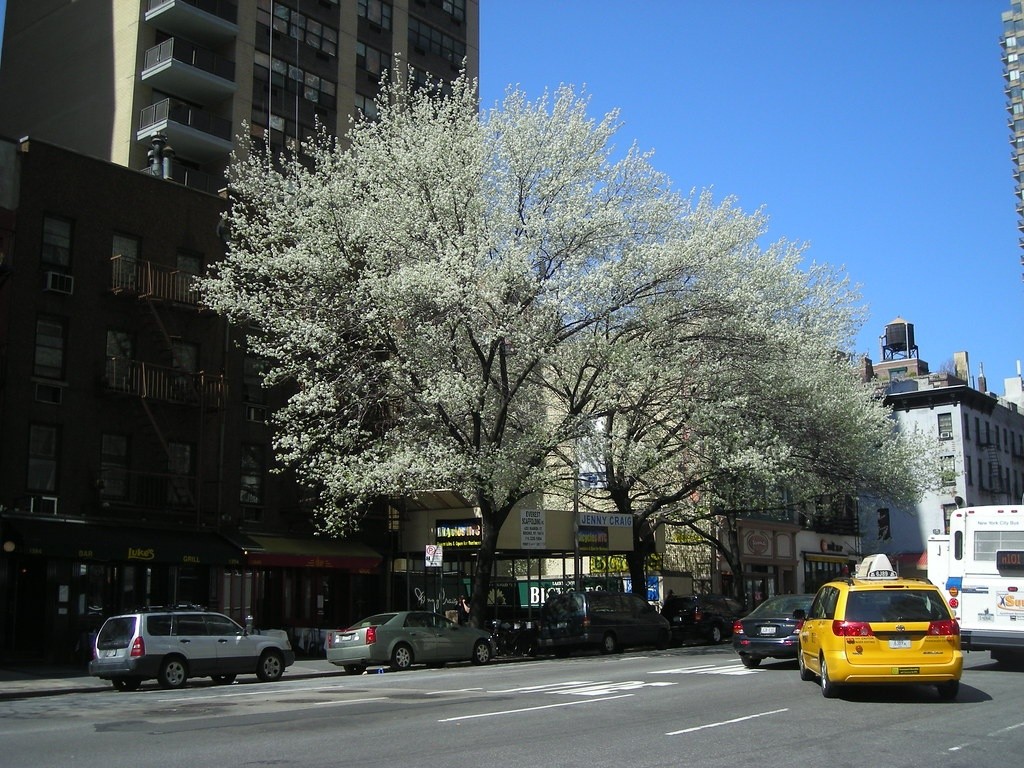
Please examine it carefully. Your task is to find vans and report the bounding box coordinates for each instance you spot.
[538,590,674,654]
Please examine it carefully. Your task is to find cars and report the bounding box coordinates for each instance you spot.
[660,593,738,648]
[325,610,498,673]
[793,553,963,700]
[733,594,816,669]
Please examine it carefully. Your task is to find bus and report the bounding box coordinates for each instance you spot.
[944,503,1024,663]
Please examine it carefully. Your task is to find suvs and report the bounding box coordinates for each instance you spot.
[88,605,297,693]
